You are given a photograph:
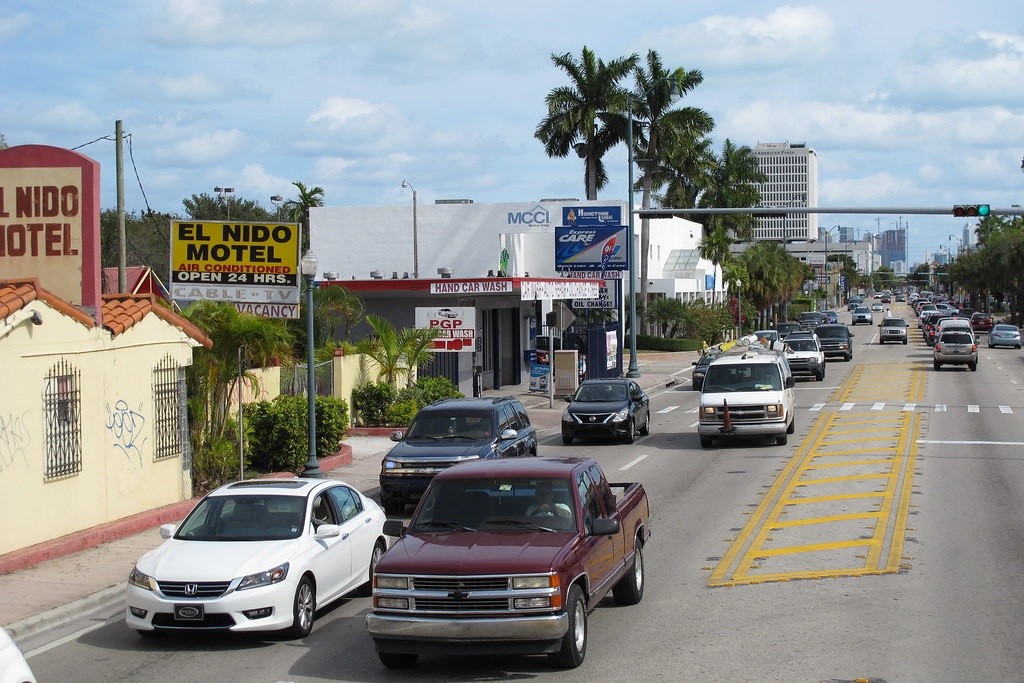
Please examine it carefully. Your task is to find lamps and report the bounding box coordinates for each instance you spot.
[649,281,654,284]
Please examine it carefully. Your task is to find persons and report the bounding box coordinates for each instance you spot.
[886,308,891,317]
[526,481,572,519]
[702,341,708,349]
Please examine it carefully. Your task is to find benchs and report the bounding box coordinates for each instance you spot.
[489,495,536,516]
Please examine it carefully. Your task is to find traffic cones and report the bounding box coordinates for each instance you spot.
[718,398,737,433]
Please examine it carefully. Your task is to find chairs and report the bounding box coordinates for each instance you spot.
[225,503,260,531]
[456,491,491,522]
[551,491,576,530]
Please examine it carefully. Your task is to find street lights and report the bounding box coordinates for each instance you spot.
[402,179,418,278]
[735,278,742,338]
[825,225,856,310]
[626,74,681,378]
[301,249,324,478]
[783,194,806,320]
[930,235,962,270]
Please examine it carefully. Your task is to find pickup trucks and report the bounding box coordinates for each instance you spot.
[365,458,652,669]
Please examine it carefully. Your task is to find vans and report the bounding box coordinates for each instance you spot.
[698,349,795,446]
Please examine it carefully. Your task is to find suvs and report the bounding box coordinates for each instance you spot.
[379,396,538,513]
[847,299,909,345]
[776,312,856,381]
[933,331,979,372]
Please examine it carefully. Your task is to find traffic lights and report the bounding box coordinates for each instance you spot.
[953,204,990,217]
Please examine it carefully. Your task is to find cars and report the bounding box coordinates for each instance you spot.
[822,311,838,322]
[126,478,392,638]
[754,331,784,349]
[562,377,651,444]
[872,289,1022,349]
[691,352,716,391]
